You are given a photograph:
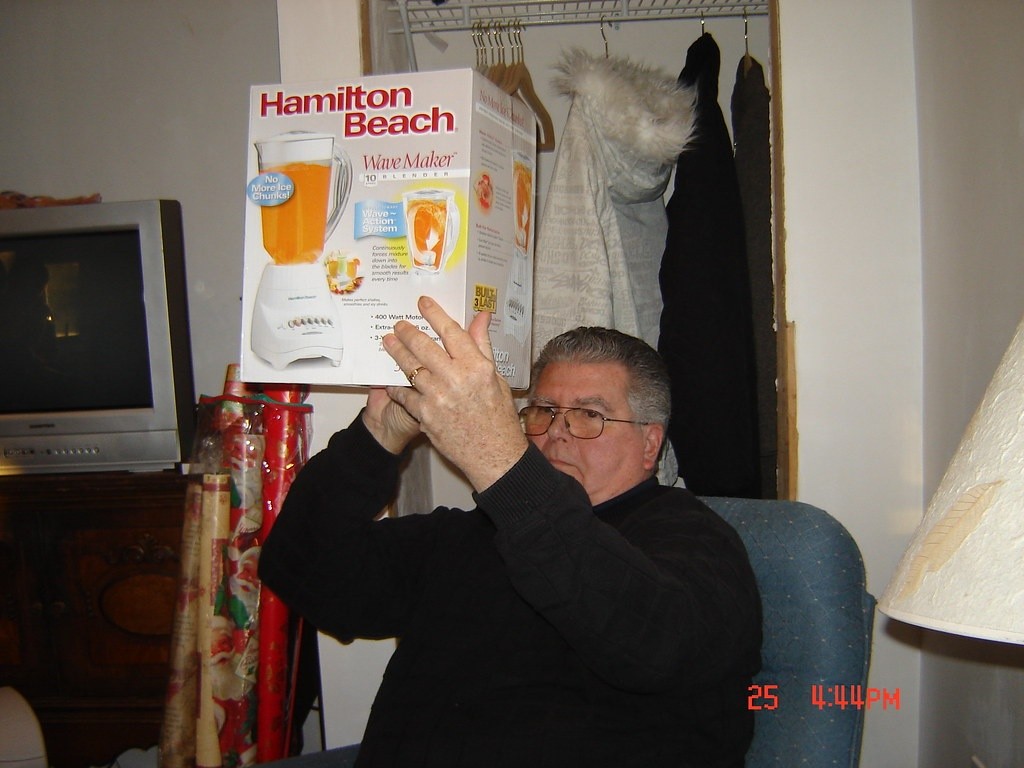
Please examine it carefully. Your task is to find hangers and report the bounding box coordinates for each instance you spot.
[742,9,751,58]
[471,20,555,153]
[600,14,612,59]
[699,10,705,35]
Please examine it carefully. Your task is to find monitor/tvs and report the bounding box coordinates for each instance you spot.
[0,198,196,476]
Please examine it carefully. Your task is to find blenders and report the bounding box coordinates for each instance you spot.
[250,129,354,369]
[503,149,535,344]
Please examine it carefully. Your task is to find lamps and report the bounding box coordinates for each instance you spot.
[876,316,1024,645]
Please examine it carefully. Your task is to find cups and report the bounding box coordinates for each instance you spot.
[329,257,339,277]
[347,261,357,277]
[337,256,347,275]
[405,190,454,270]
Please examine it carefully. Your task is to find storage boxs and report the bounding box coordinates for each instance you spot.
[239,67,536,391]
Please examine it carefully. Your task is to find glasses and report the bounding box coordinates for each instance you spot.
[517,405,648,439]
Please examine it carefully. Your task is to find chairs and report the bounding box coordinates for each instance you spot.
[253,494,876,768]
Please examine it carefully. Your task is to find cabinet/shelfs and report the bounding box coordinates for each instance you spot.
[0,470,202,768]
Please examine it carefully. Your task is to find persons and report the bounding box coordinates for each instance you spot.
[250,295,762,768]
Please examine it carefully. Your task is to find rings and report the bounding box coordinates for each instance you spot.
[409,366,426,386]
